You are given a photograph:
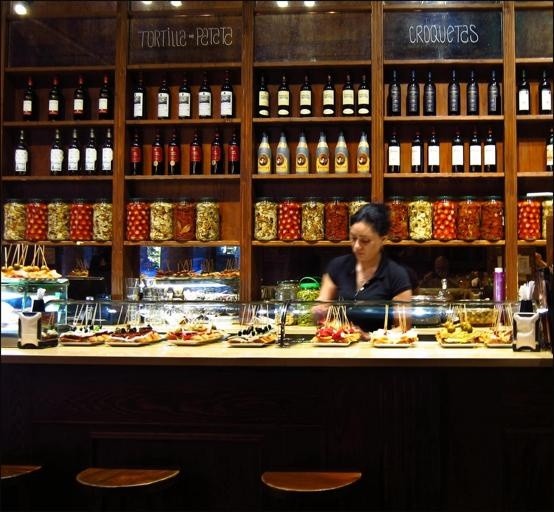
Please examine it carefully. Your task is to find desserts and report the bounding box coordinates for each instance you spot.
[59,325,514,343]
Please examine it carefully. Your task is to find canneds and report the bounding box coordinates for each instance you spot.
[518,193,554,241]
[3,197,112,243]
[124,195,221,240]
[253,196,371,242]
[385,194,505,241]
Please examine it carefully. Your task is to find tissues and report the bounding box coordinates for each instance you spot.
[512,280,541,352]
[17,287,59,349]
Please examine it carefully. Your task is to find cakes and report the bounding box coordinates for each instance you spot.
[1,242,63,280]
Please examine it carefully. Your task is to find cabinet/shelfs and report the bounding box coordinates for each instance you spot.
[1,65,114,301]
[250,59,373,313]
[381,58,513,306]
[117,65,248,304]
[515,59,554,306]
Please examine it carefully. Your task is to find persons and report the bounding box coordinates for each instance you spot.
[310,203,412,342]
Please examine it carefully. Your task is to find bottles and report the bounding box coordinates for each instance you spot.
[546,128,554,172]
[388,129,497,172]
[128,129,242,175]
[14,127,30,176]
[47,74,65,122]
[157,77,171,120]
[256,130,371,173]
[407,69,420,116]
[323,73,335,116]
[389,70,401,116]
[466,70,480,116]
[133,79,146,119]
[300,73,313,117]
[423,70,436,116]
[488,70,501,115]
[341,72,356,115]
[438,260,508,309]
[97,73,113,120]
[357,74,371,116]
[256,72,270,117]
[72,76,90,121]
[21,75,39,123]
[178,79,192,119]
[448,70,460,116]
[50,127,113,176]
[539,70,550,116]
[221,71,236,118]
[83,297,95,328]
[277,73,291,117]
[198,72,213,119]
[516,68,531,115]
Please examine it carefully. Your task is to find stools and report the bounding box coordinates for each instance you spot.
[260,470,364,511]
[0,462,43,512]
[76,464,181,512]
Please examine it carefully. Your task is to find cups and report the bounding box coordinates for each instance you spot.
[126,277,158,302]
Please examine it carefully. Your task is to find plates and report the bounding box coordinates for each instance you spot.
[158,276,240,281]
[64,335,514,351]
[4,274,63,282]
